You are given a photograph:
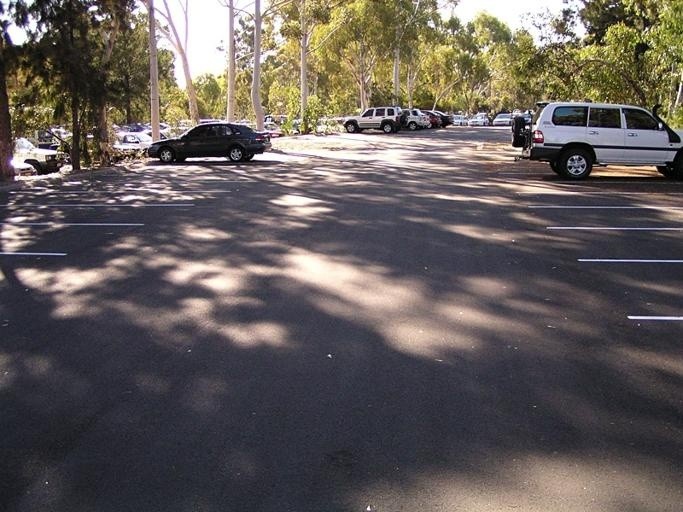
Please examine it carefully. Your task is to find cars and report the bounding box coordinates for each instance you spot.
[402,108,536,131]
[10,136,65,175]
[147,122,271,163]
[10,161,37,177]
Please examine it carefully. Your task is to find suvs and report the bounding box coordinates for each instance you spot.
[342,105,407,134]
[511,101,682,181]
